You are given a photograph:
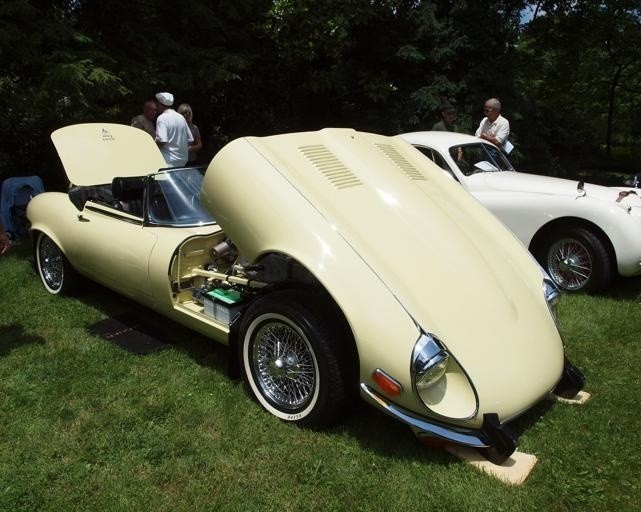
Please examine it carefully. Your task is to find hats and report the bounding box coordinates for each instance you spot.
[155,92,175,107]
[438,103,455,113]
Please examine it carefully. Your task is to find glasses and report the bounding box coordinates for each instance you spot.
[484,108,495,112]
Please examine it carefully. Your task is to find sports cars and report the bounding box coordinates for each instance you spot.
[390,128,641,291]
[25,118,589,463]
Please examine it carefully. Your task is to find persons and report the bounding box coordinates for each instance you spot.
[1,222,10,257]
[430,105,458,132]
[474,97,514,157]
[455,147,474,174]
[177,103,204,164]
[130,98,157,139]
[156,89,193,166]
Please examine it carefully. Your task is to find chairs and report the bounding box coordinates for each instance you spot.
[150,180,173,221]
[113,177,143,220]
